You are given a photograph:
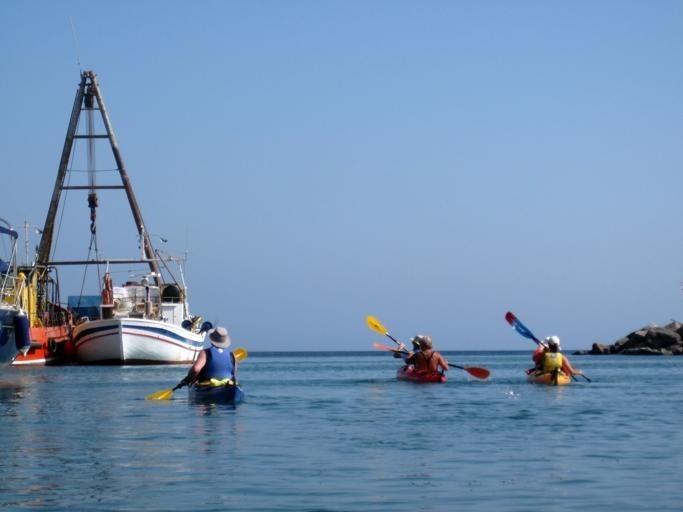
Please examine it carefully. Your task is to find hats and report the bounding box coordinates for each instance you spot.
[419,336,431,346]
[548,336,560,345]
[410,335,424,346]
[209,327,231,349]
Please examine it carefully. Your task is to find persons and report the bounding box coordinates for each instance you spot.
[180,325,237,386]
[532,335,582,376]
[393,334,424,366]
[404,336,449,372]
[536,337,570,374]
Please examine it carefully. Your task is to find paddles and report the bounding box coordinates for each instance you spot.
[373,343,489,379]
[144,347,247,401]
[505,311,592,383]
[368,315,408,351]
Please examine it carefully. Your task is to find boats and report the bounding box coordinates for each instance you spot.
[71,221,206,365]
[0,217,72,365]
[527,369,570,385]
[188,381,241,403]
[397,365,446,383]
